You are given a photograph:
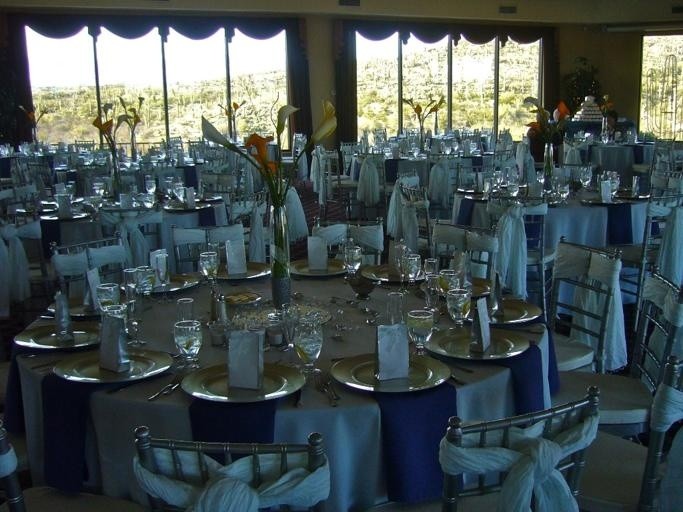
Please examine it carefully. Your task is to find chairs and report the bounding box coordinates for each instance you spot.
[399,184,432,255]
[508,205,555,324]
[130,427,331,511]
[288,129,535,218]
[427,153,461,207]
[440,384,600,512]
[50,242,88,301]
[44,242,87,307]
[318,146,364,218]
[464,226,498,283]
[602,216,671,333]
[346,221,381,266]
[199,170,236,205]
[86,232,126,285]
[170,224,208,274]
[312,216,346,260]
[396,169,420,186]
[559,357,683,512]
[547,237,623,369]
[491,198,557,323]
[463,226,499,304]
[546,267,681,423]
[431,219,466,269]
[207,221,245,252]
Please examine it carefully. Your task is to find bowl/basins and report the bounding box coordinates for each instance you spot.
[348,279,378,300]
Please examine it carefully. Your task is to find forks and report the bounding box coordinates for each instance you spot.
[320,370,340,401]
[147,375,181,401]
[314,375,337,407]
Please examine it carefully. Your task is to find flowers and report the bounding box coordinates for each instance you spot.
[405,96,445,147]
[218,98,244,138]
[201,95,338,280]
[20,105,58,130]
[116,96,144,155]
[92,105,120,202]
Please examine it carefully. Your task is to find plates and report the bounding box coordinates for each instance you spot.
[224,292,261,305]
[424,326,531,360]
[216,261,272,279]
[48,296,100,317]
[14,324,100,349]
[419,276,492,298]
[331,353,451,393]
[120,273,199,292]
[465,195,488,202]
[614,190,651,201]
[487,297,543,328]
[582,195,635,205]
[362,263,425,282]
[457,186,482,194]
[181,362,305,403]
[290,258,347,277]
[163,203,213,212]
[53,349,173,384]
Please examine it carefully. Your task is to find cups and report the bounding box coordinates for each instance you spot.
[138,268,154,296]
[402,254,422,287]
[177,298,194,320]
[143,194,155,209]
[120,194,128,209]
[384,148,390,160]
[296,317,323,376]
[282,302,298,368]
[58,195,73,220]
[97,282,120,328]
[103,306,124,317]
[93,186,99,196]
[174,321,203,373]
[344,247,362,280]
[470,143,476,154]
[440,270,458,291]
[445,146,451,155]
[207,321,225,346]
[393,245,410,296]
[268,326,283,346]
[408,310,433,357]
[558,184,569,204]
[580,174,591,192]
[452,142,458,154]
[248,326,265,345]
[412,148,419,160]
[447,289,472,328]
[425,274,440,309]
[156,253,171,304]
[425,259,437,276]
[165,177,176,202]
[385,292,404,325]
[123,268,137,287]
[208,241,221,272]
[602,135,609,145]
[200,251,217,286]
[126,283,142,298]
[144,176,157,194]
[126,300,146,348]
[495,171,502,188]
[508,181,520,206]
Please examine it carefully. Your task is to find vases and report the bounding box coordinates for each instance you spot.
[110,151,120,202]
[31,128,38,148]
[269,203,292,312]
[130,130,138,161]
